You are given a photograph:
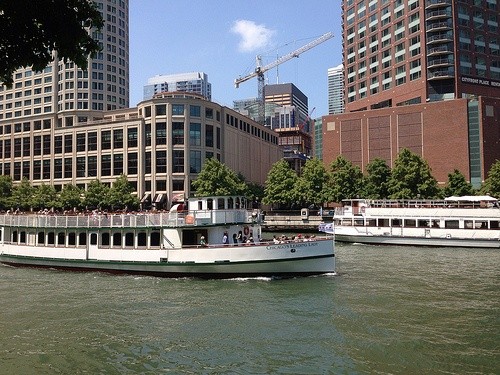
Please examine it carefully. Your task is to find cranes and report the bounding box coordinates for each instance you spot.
[234,31,335,126]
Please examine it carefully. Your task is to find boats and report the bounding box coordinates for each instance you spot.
[318,195,500,248]
[0,194,337,277]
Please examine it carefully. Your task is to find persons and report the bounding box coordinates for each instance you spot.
[201,230,317,247]
[0,207,169,227]
